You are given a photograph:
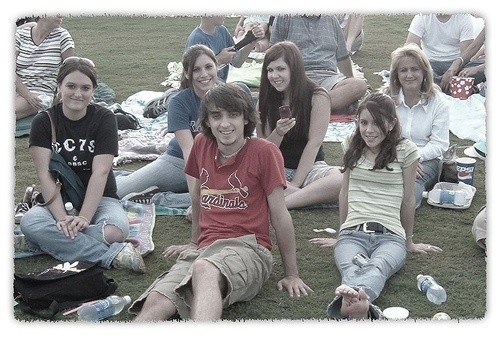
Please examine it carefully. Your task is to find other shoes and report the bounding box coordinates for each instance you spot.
[15,201,32,224]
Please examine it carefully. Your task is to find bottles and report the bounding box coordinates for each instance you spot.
[422,189,466,207]
[416,274,447,305]
[64,202,79,216]
[77,295,131,322]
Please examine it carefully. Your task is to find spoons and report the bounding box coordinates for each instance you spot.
[313,228,336,233]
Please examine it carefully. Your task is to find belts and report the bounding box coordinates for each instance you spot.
[348,222,392,234]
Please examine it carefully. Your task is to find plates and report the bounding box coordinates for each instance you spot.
[426,182,477,209]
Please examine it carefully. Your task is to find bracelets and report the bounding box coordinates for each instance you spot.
[191,241,197,245]
[457,57,464,66]
[276,129,282,136]
[79,216,90,224]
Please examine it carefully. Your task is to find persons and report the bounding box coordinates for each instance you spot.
[406,13,486,93]
[142,15,265,119]
[15,15,74,118]
[256,41,343,209]
[233,16,269,51]
[309,91,442,319]
[128,83,314,324]
[269,15,365,110]
[20,56,146,272]
[337,14,365,54]
[284,45,450,209]
[116,44,218,221]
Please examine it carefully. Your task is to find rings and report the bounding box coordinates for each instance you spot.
[428,246,430,248]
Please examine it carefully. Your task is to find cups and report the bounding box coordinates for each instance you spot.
[38,95,51,114]
[456,157,476,185]
[449,76,475,100]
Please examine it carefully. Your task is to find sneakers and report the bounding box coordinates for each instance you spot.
[112,242,146,273]
[121,185,159,205]
[142,88,179,118]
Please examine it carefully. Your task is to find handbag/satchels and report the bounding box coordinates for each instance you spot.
[34,111,86,211]
[114,108,139,129]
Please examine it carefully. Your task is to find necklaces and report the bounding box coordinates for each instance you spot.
[220,139,246,158]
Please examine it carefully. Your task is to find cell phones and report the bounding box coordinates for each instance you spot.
[279,105,293,119]
